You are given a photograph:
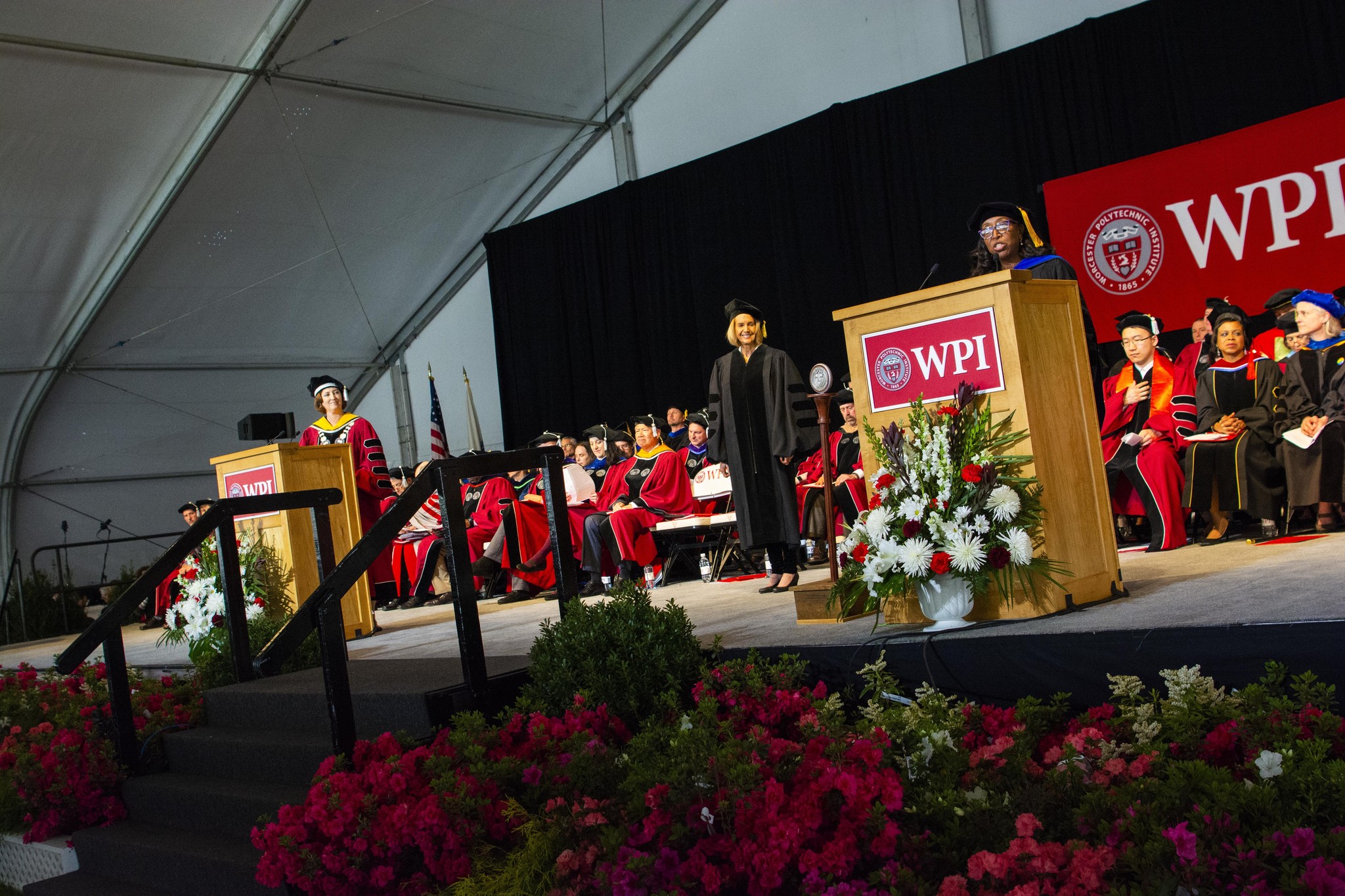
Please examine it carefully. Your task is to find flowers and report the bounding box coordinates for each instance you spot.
[247,635,1345,896]
[154,520,296,687]
[825,378,1077,636]
[0,651,212,842]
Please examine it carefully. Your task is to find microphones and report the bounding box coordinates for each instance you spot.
[61,521,67,533]
[918,264,940,291]
[265,430,286,446]
[99,519,111,531]
[993,253,1000,272]
[290,431,300,442]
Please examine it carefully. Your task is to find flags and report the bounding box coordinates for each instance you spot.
[428,377,450,460]
[464,379,486,451]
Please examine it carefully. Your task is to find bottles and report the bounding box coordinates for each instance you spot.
[601,576,612,591]
[699,553,711,583]
[644,564,656,589]
[805,539,815,560]
[478,581,486,598]
[1261,518,1277,538]
[764,551,772,578]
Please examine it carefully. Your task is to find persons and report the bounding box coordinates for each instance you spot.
[966,202,1106,434]
[705,299,821,594]
[1101,284,1345,553]
[299,375,396,634]
[139,498,218,630]
[795,373,869,565]
[381,404,739,611]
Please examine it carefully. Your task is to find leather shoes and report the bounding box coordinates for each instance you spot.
[424,591,453,606]
[396,595,426,609]
[139,615,164,630]
[578,581,606,597]
[602,579,634,597]
[807,546,829,565]
[382,598,408,611]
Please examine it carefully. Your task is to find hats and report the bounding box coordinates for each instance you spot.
[389,465,414,489]
[195,499,217,506]
[582,413,710,450]
[1114,282,1345,334]
[832,388,854,406]
[724,299,767,338]
[178,503,196,513]
[966,202,1044,249]
[527,430,562,448]
[458,449,488,458]
[307,375,348,403]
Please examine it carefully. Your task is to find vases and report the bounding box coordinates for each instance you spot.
[912,571,977,632]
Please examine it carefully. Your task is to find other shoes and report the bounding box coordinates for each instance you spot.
[472,557,495,579]
[497,589,531,604]
[516,559,547,573]
[544,592,558,601]
[1117,503,1345,554]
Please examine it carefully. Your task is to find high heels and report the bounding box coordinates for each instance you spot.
[759,576,781,594]
[772,572,799,593]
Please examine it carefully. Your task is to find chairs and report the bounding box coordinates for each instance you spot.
[656,463,733,588]
[710,510,807,581]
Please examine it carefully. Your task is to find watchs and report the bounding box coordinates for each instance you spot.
[468,516,475,528]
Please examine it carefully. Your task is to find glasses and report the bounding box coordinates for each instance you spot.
[1121,334,1154,347]
[618,444,630,450]
[393,483,404,492]
[977,220,1020,240]
[562,443,575,447]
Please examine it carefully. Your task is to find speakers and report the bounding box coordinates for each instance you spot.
[237,411,295,440]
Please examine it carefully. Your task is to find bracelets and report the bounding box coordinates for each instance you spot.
[1211,425,1218,434]
